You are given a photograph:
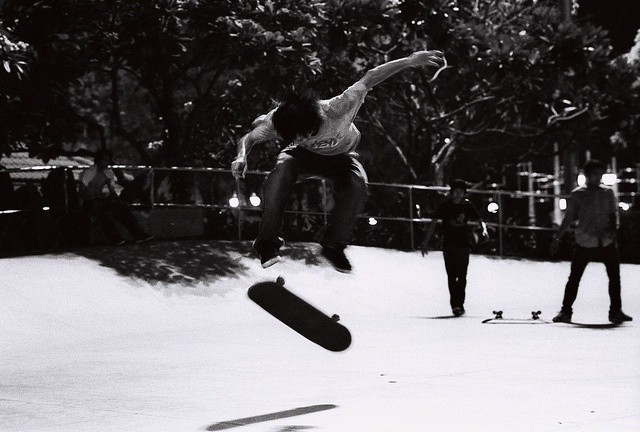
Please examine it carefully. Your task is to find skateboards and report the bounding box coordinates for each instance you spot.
[248,275,351,352]
[551,316,627,331]
[484,310,555,325]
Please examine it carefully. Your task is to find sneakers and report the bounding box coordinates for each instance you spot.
[255,242,281,269]
[609,310,632,322]
[321,246,351,273]
[553,311,573,322]
[453,307,462,315]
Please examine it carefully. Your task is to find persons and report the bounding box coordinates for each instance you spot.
[232,50,444,274]
[548,160,634,322]
[420,179,489,315]
[77,150,156,249]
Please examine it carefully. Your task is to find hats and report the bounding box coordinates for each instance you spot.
[450,179,466,189]
[584,160,608,175]
